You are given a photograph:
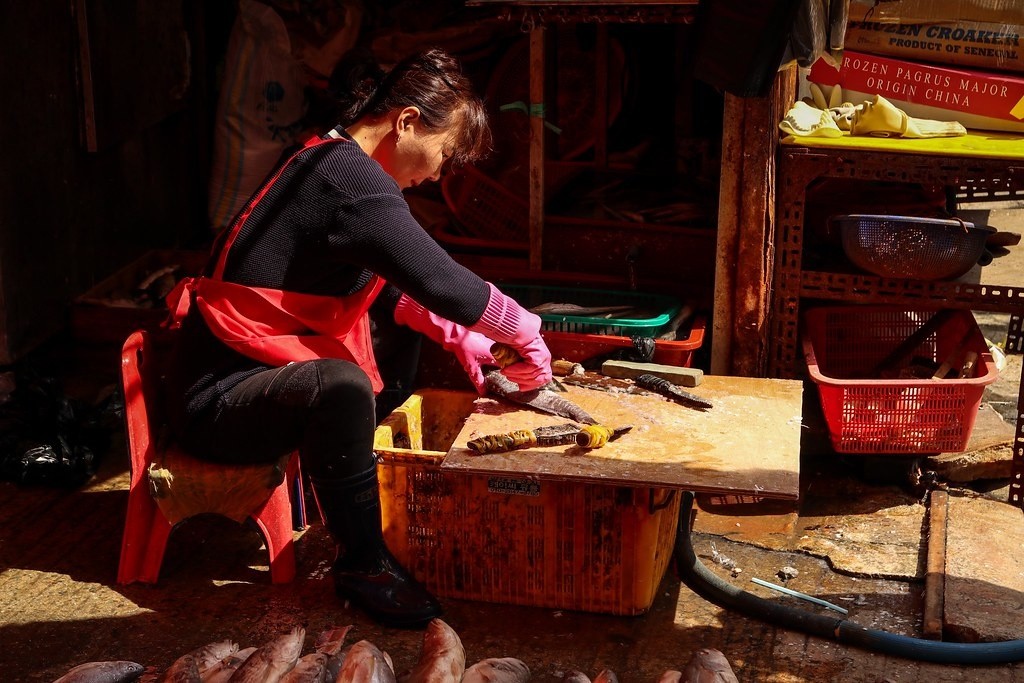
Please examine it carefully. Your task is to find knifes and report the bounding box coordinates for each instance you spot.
[552,358,652,395]
[636,374,713,408]
[490,343,567,392]
[576,424,633,448]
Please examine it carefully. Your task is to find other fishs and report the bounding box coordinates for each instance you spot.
[484,369,603,427]
[52,618,739,683]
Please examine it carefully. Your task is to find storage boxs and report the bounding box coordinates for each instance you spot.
[829,0,1024,71]
[805,48,1024,134]
[371,382,681,612]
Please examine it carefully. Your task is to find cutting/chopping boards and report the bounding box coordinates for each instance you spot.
[440,370,803,501]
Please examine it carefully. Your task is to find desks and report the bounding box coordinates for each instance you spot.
[773,133,1024,506]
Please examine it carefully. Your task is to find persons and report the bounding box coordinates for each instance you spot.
[143,50,552,630]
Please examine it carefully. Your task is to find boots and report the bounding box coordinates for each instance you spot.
[317,465,444,629]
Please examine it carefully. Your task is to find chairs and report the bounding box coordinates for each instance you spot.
[110,329,326,583]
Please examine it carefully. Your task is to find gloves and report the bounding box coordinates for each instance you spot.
[464,280,552,393]
[393,291,502,398]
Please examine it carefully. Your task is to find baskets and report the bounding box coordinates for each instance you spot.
[831,211,996,280]
